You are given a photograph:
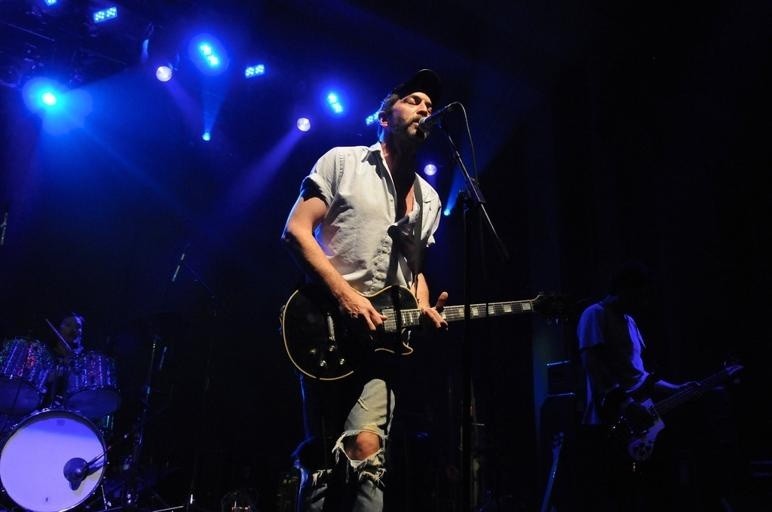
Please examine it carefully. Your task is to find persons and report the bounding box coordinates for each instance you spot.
[569,271,703,512]
[40,308,87,406]
[270,62,452,511]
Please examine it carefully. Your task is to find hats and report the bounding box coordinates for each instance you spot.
[392,68,441,105]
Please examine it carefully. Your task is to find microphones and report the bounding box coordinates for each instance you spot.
[419,101,458,132]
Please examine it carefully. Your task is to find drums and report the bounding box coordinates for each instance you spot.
[65,352,120,417]
[1,408,107,511]
[0,337,53,411]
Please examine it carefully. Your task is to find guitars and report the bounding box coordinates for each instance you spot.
[282,287,540,381]
[612,363,742,460]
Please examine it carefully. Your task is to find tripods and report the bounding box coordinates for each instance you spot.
[148,305,219,512]
